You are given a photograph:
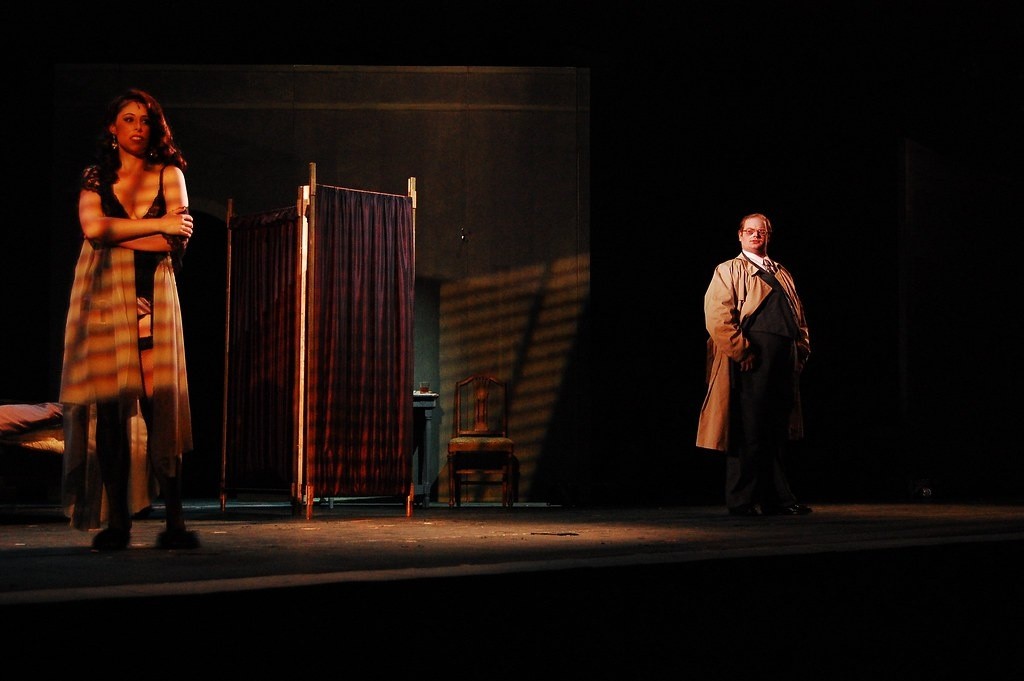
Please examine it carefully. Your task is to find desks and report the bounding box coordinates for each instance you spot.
[413,391,438,507]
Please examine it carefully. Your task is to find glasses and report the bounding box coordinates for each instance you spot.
[743,228,768,235]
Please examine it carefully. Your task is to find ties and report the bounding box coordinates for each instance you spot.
[763,260,775,275]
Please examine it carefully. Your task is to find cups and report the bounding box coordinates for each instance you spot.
[420,382,430,394]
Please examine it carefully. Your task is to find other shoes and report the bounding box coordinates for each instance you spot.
[775,503,812,516]
[728,504,763,519]
[91,524,132,552]
[157,529,200,551]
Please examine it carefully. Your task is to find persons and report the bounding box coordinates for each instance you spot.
[693,213,815,518]
[59,83,207,553]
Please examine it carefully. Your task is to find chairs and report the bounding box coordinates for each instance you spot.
[449,374,517,512]
